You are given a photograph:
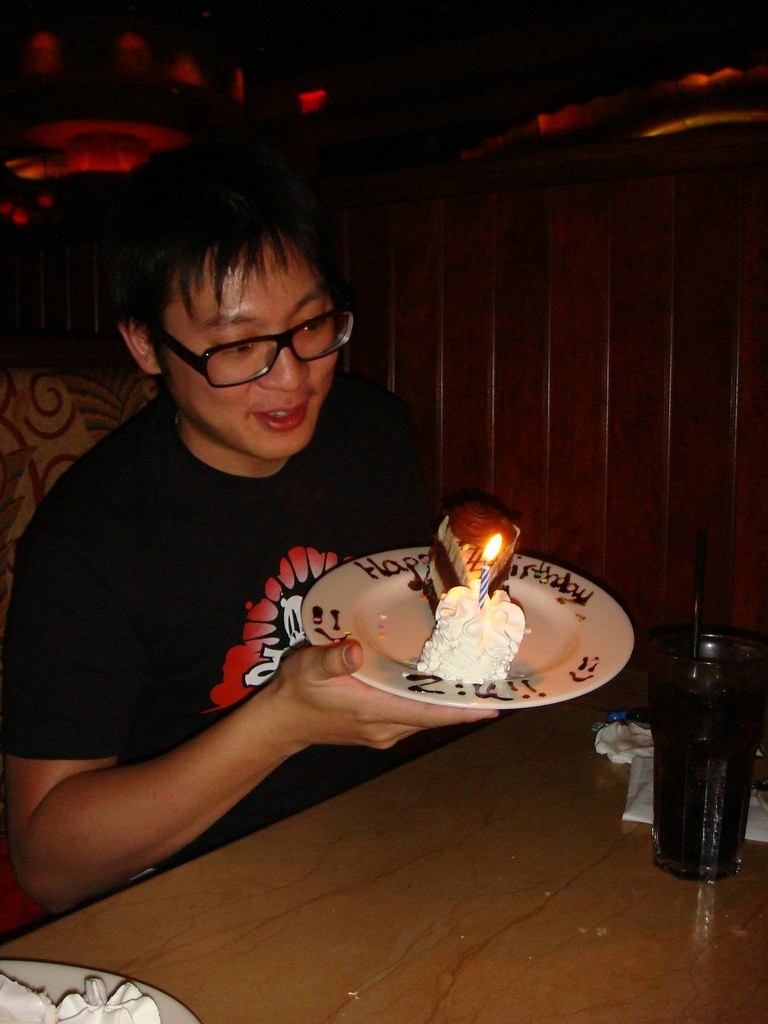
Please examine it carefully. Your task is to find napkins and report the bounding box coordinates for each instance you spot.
[622,757,768,843]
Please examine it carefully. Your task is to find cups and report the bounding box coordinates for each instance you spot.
[641,629,768,883]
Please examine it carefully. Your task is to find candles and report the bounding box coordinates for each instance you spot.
[478,534,501,611]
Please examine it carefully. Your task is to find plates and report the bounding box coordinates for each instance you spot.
[300,547,634,712]
[0,960,200,1024]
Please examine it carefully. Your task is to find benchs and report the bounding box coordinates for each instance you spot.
[0,364,159,835]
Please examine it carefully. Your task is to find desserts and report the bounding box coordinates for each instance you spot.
[0,973,162,1024]
[416,499,526,684]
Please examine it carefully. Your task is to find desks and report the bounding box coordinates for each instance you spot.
[0,636,768,1024]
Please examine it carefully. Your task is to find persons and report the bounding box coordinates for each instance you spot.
[0,146,500,918]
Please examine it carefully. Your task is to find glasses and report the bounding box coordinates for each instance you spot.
[146,299,355,388]
